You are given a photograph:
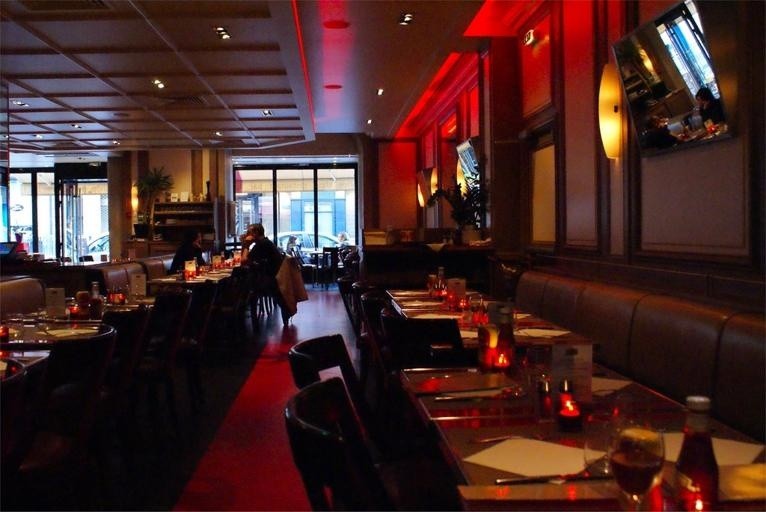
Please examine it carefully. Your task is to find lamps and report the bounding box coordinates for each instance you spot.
[598,64,627,159]
[456,159,468,201]
[431,168,438,196]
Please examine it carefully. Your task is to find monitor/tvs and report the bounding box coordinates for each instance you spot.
[455,136,480,181]
[608,0,736,159]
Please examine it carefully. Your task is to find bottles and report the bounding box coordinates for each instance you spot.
[184,250,242,281]
[448,296,582,432]
[676,397,718,511]
[105,284,131,305]
[433,267,445,301]
[91,282,102,320]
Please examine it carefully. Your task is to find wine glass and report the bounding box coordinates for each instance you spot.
[608,419,665,512]
[427,274,436,297]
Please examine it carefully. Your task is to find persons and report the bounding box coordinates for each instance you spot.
[10,232,29,256]
[695,87,728,127]
[638,113,677,149]
[167,229,206,275]
[285,235,300,258]
[332,231,355,266]
[241,224,283,290]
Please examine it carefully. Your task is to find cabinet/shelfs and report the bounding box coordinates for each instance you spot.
[150,197,220,256]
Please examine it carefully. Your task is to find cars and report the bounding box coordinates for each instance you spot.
[267,232,341,258]
[87,234,109,254]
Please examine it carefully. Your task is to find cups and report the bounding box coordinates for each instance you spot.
[46,288,66,318]
[6,313,24,340]
[318,254,323,268]
[101,255,107,262]
[324,252,331,268]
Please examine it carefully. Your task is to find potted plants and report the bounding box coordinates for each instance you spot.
[427,157,487,244]
